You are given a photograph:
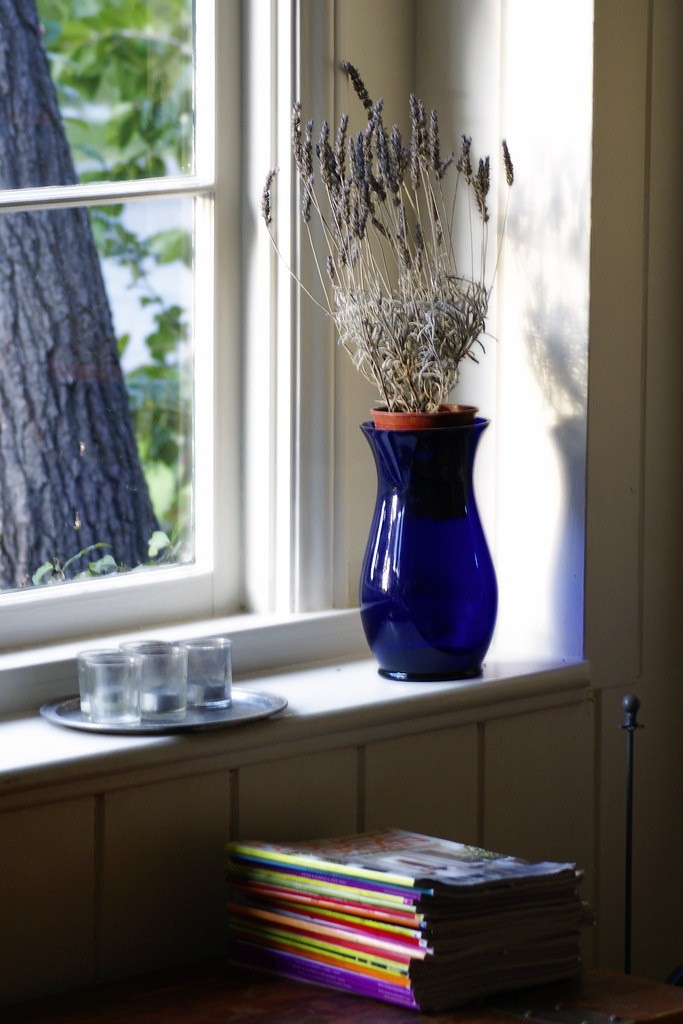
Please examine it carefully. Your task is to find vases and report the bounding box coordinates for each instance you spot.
[355,403,499,683]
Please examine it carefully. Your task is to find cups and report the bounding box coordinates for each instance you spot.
[120,641,169,653]
[78,649,124,714]
[88,655,142,726]
[138,645,188,721]
[182,638,233,710]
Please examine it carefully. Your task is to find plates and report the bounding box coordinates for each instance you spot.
[39,685,287,730]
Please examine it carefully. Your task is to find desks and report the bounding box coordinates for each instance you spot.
[1,932,682,1024]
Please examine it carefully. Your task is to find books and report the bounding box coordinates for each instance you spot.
[223,821,595,1014]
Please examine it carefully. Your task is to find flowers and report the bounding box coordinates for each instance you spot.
[260,64,515,411]
